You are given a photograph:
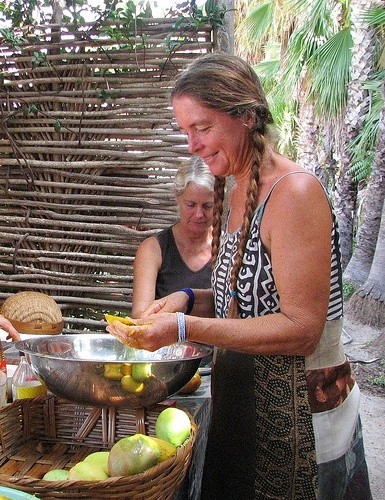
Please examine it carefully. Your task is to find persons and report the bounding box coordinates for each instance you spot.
[130,156,218,369]
[0,313,21,345]
[102,52,373,500]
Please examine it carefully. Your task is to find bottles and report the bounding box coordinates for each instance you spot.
[12,350,47,401]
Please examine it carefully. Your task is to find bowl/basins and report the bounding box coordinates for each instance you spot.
[15,333,213,409]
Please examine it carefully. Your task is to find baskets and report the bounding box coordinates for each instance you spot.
[0,395,197,500]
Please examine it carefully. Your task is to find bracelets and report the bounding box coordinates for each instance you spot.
[181,287,196,317]
[173,310,187,345]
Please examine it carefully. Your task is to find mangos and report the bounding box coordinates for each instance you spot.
[181,370,201,394]
[103,363,154,392]
[42,408,191,482]
